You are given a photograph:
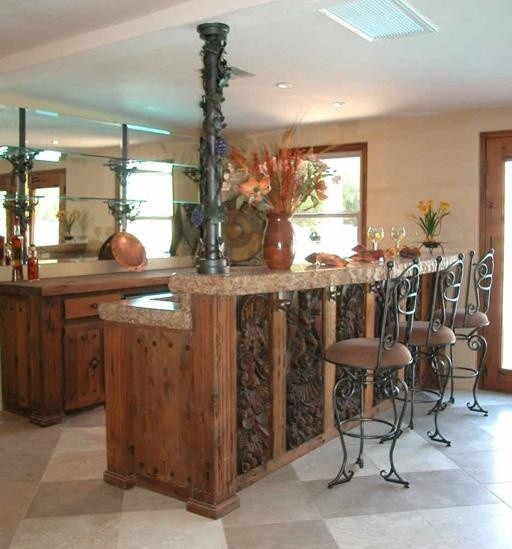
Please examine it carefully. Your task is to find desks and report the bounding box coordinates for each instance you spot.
[98,253,465,518]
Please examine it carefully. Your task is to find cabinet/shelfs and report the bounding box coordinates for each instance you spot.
[1,262,254,429]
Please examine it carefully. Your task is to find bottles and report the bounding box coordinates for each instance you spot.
[5,217,39,282]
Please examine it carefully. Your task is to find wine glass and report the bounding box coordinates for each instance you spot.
[367,224,405,265]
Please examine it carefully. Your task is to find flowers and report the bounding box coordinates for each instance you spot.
[407,200,452,241]
[226,118,344,217]
[56,208,81,237]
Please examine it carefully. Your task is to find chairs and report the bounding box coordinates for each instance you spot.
[432,249,495,417]
[378,252,464,448]
[323,255,420,489]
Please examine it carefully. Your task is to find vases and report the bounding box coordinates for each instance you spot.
[262,210,297,269]
[416,233,447,255]
[62,222,72,242]
